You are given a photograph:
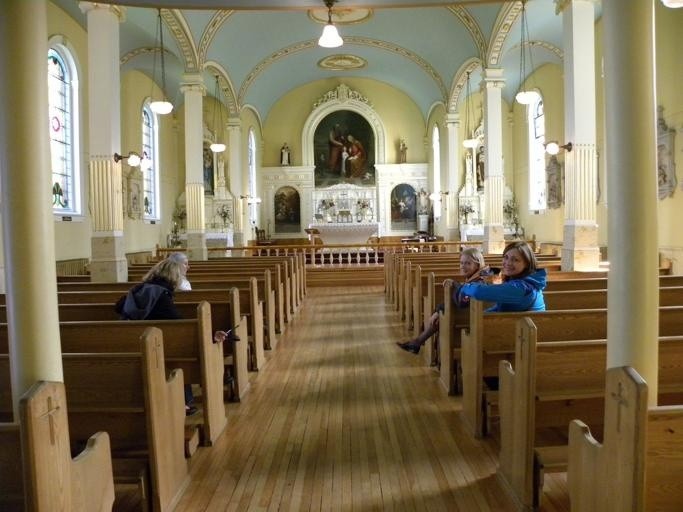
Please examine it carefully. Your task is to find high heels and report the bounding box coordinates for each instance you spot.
[396,341,421,354]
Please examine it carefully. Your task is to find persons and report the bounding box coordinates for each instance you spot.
[280,142,291,165]
[396,247,493,355]
[329,120,372,180]
[400,139,408,162]
[168,253,191,291]
[114,259,234,416]
[458,239,547,390]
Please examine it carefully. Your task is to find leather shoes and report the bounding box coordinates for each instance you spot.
[186,407,197,416]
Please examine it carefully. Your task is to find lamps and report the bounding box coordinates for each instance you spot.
[462,71,478,148]
[515,0,540,105]
[149,7,173,115]
[210,76,226,153]
[546,142,572,155]
[318,0,344,48]
[114,151,143,167]
